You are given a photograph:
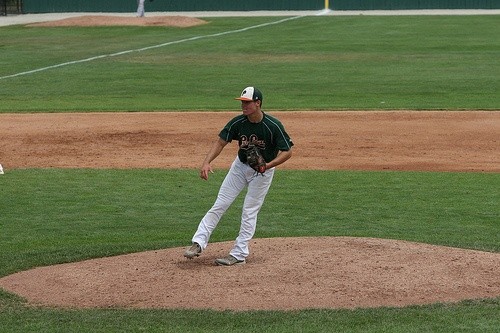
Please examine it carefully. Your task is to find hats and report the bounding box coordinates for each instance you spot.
[233,86,262,102]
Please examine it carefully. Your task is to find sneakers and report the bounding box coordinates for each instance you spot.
[215,253,246,267]
[183,242,202,260]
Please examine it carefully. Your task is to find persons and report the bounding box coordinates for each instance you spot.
[183,86,294,266]
